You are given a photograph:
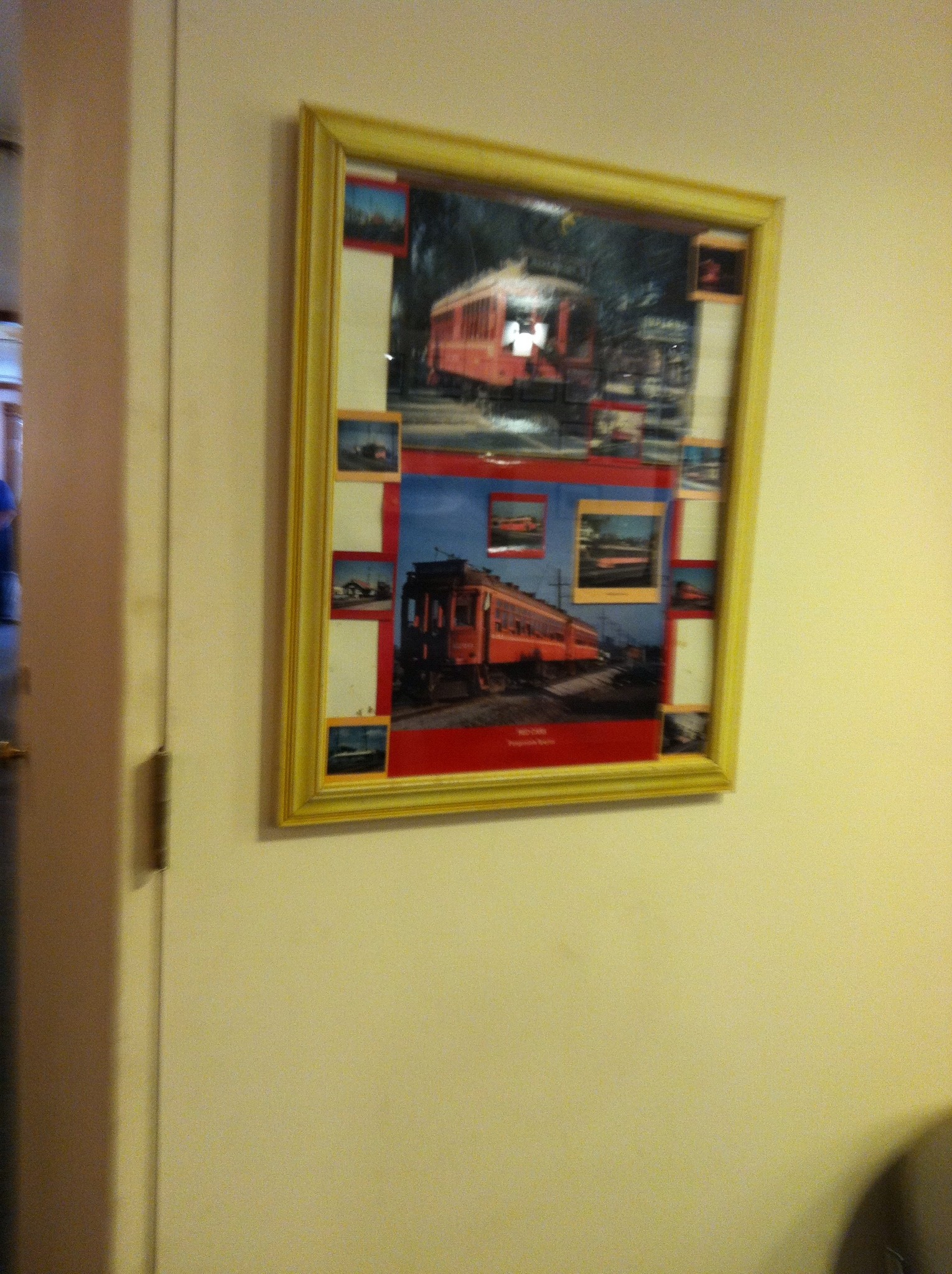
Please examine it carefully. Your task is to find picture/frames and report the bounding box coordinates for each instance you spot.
[270,98,790,830]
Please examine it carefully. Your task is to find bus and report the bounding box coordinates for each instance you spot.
[495,516,538,534]
[611,425,634,441]
[363,444,387,460]
[598,543,653,571]
[674,579,708,602]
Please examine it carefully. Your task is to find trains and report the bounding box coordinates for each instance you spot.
[428,254,602,404]
[400,557,605,698]
